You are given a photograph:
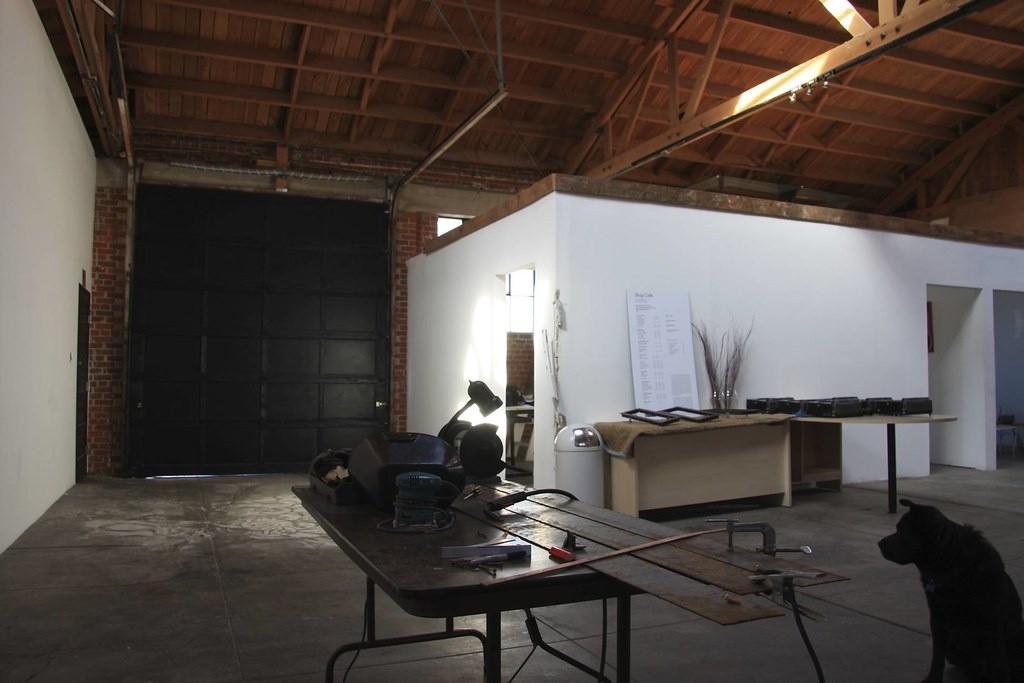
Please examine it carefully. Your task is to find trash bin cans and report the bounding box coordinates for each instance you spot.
[553,422,605,507]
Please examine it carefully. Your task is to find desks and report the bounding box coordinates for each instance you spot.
[291,484,713,683]
[791,413,958,513]
[996,423,1020,460]
[587,413,796,518]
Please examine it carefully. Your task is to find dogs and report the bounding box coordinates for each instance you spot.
[878,498,1024,683]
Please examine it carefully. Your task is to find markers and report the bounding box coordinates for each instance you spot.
[471,550,527,565]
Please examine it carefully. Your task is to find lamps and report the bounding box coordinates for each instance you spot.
[788,92,797,104]
[437,379,503,437]
[821,79,831,88]
[805,87,813,97]
